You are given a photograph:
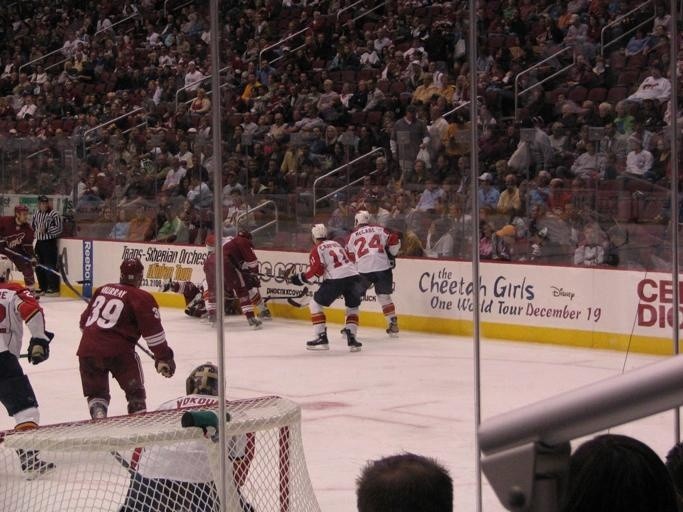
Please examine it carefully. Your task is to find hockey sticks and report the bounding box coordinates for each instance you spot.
[57,254,156,359]
[287,297,311,308]
[228,253,324,285]
[4,246,90,284]
[226,287,309,300]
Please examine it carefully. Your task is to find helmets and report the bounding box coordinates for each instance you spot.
[311,223,329,238]
[0,254,14,283]
[354,210,370,227]
[119,258,144,287]
[238,230,250,239]
[185,362,225,397]
[14,204,29,213]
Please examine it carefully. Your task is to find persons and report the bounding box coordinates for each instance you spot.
[67,0,204,244]
[564,432,673,512]
[0,253,54,479]
[78,259,177,418]
[289,224,364,353]
[27,195,65,293]
[207,0,262,228]
[122,363,254,511]
[356,454,455,512]
[405,1,683,273]
[344,1,403,209]
[0,204,36,295]
[206,231,262,330]
[345,210,402,339]
[264,0,343,224]
[1,0,66,198]
[160,269,273,322]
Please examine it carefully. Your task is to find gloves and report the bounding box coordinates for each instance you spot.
[27,331,54,366]
[290,271,310,287]
[152,348,176,378]
[384,247,396,267]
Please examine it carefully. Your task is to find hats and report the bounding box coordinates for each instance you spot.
[38,195,49,203]
[477,172,495,183]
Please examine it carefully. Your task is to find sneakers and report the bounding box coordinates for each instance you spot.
[340,327,346,334]
[305,327,330,346]
[385,316,399,334]
[207,312,217,323]
[247,316,263,327]
[256,307,272,320]
[34,286,46,294]
[45,288,59,294]
[346,326,363,348]
